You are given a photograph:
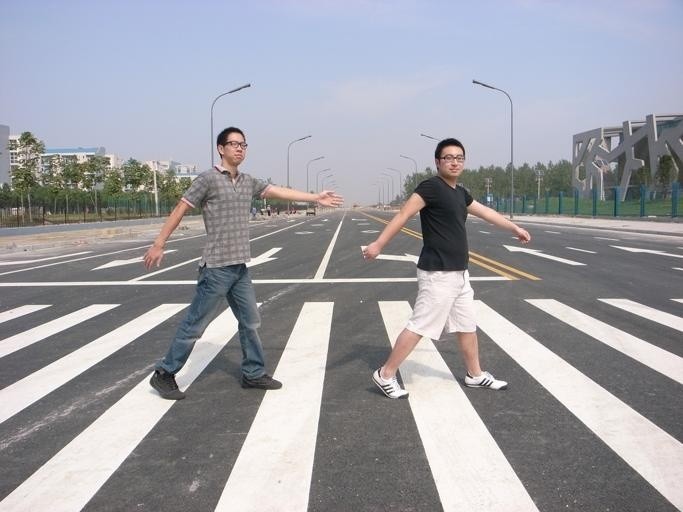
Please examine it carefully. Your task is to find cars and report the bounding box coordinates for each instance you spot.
[304,207,316,216]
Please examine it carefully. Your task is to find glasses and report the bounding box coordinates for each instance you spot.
[443,153,467,164]
[230,139,249,151]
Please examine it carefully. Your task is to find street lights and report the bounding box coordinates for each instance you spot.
[472,81,515,220]
[482,177,491,194]
[284,135,311,218]
[208,83,251,169]
[534,170,545,199]
[399,154,419,191]
[419,133,441,144]
[305,156,340,215]
[372,167,404,211]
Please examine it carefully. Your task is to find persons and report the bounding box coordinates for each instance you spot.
[250,205,300,220]
[144,126,345,400]
[362,138,532,400]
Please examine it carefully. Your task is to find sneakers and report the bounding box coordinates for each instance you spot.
[464,369,508,391]
[149,368,186,400]
[371,365,410,401]
[239,373,283,391]
